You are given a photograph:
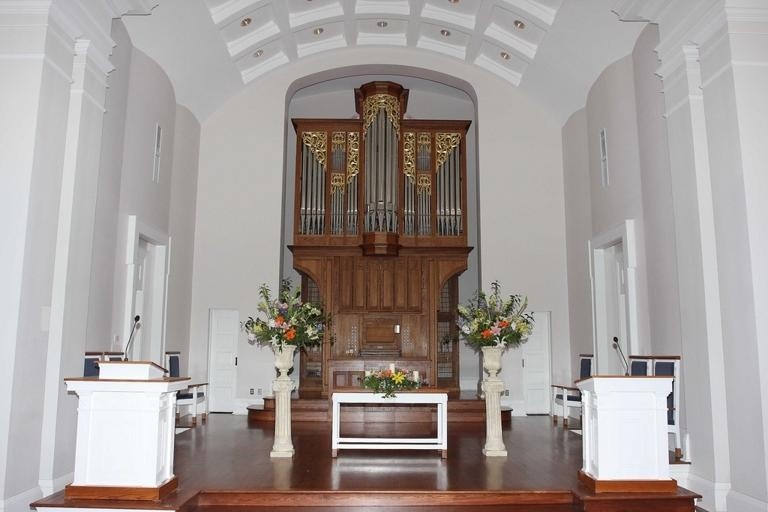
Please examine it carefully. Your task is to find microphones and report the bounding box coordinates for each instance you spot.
[613,337,628,375]
[124,316,140,362]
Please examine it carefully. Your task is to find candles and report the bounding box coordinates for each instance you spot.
[389,362,395,373]
[413,371,419,378]
[365,371,371,377]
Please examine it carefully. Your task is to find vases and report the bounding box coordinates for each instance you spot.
[481,346,506,382]
[271,345,297,381]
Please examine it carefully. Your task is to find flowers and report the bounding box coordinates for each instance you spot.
[440,279,537,350]
[238,276,337,354]
[362,367,419,399]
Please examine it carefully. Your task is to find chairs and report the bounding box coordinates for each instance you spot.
[550,354,684,463]
[83,350,209,430]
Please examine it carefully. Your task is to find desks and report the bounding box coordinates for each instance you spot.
[331,387,448,459]
